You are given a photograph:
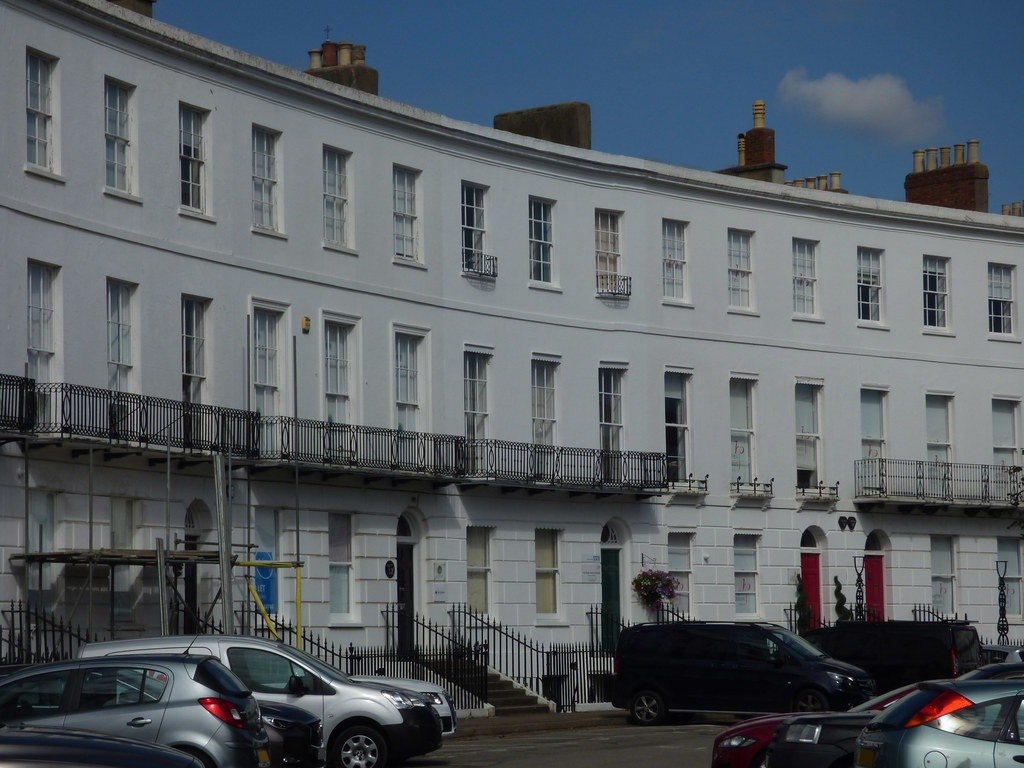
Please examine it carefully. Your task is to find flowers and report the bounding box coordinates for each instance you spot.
[633,568,683,609]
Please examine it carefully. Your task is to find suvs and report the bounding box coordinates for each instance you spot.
[776,618,985,699]
[610,618,879,729]
[68,635,444,768]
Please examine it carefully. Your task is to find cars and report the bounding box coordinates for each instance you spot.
[709,644,1024,768]
[346,666,458,741]
[0,653,274,768]
